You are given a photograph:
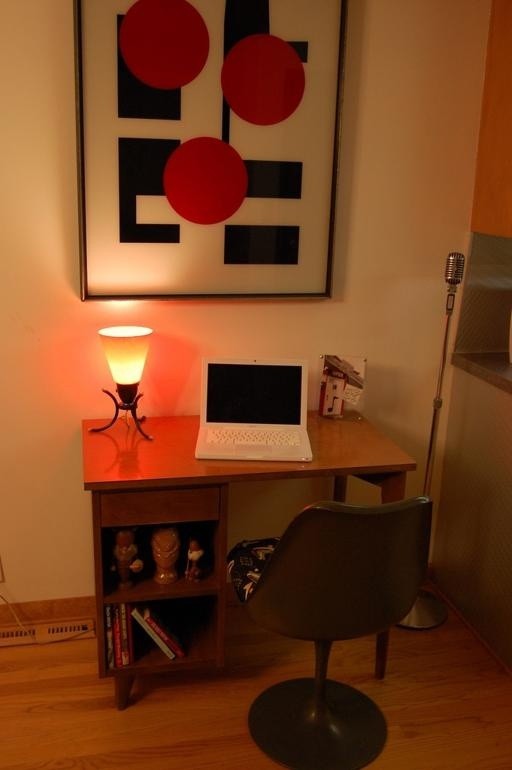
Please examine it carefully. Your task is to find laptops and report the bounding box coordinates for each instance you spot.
[194,355,314,463]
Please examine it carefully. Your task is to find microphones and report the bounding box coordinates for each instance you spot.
[444,253,464,317]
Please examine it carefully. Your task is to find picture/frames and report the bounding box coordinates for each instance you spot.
[74,0,346,299]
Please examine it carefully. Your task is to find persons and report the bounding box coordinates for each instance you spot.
[184,539,205,584]
[109,531,146,591]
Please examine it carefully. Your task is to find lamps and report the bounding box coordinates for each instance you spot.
[90,320,167,446]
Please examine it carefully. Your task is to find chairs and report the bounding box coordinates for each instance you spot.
[230,499,441,766]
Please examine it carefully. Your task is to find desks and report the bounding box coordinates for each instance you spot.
[83,413,420,710]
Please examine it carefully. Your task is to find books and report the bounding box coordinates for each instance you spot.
[105,602,186,668]
